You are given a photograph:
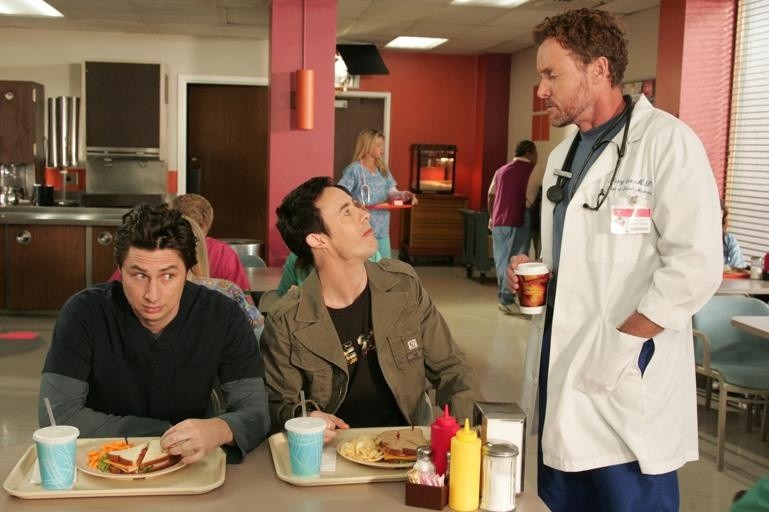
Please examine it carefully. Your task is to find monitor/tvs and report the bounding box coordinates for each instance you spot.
[336,43,389,75]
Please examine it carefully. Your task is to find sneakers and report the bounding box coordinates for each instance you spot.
[498,300,525,315]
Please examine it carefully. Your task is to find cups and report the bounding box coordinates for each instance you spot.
[34,425,79,490]
[285,417,327,479]
[394,201,402,205]
[34,185,54,206]
[514,263,549,314]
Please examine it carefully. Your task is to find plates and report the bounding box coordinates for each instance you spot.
[77,438,189,480]
[338,436,415,469]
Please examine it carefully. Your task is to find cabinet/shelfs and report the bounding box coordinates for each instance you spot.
[399,191,469,266]
[1,80,44,166]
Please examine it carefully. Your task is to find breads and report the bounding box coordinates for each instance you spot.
[375,428,430,456]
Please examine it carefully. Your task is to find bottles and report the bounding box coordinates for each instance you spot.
[416,402,520,511]
[0,161,28,206]
[749,256,763,280]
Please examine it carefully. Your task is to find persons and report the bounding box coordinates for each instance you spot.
[108,192,255,306]
[180,213,265,352]
[520,5,725,512]
[36,200,271,466]
[258,174,487,445]
[488,139,539,316]
[721,204,747,273]
[337,127,419,264]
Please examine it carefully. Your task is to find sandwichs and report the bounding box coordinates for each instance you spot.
[140,440,181,473]
[104,443,147,474]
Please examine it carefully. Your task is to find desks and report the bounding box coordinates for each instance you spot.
[711,278,769,297]
[239,267,286,293]
[730,313,768,343]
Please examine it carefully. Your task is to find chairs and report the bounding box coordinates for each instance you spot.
[238,255,266,268]
[690,294,769,475]
[258,289,281,315]
